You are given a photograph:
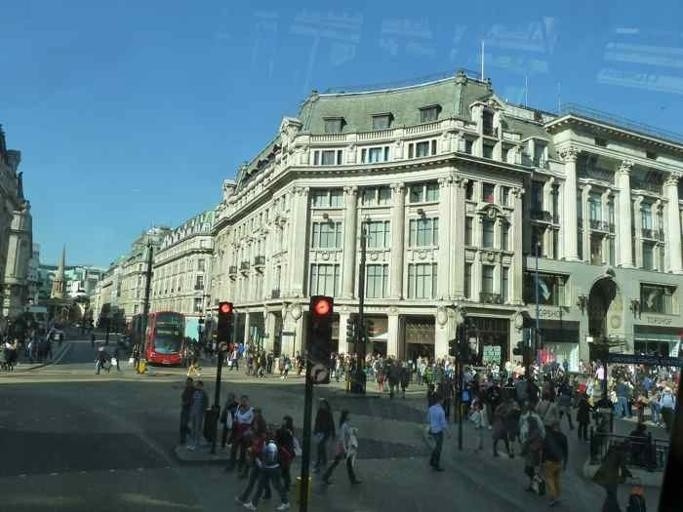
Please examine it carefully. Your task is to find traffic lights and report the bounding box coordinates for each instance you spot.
[448,338,458,358]
[345,318,356,344]
[306,293,334,365]
[512,340,525,357]
[363,318,375,344]
[216,302,232,334]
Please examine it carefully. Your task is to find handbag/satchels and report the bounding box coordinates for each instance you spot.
[225,411,232,429]
[531,473,544,496]
[285,429,302,457]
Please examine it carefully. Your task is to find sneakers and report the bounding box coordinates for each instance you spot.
[242,500,256,511]
[275,502,290,512]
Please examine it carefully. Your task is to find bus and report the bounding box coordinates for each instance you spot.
[129,311,188,368]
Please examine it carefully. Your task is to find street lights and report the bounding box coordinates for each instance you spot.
[354,233,373,377]
[139,242,154,358]
[534,240,542,330]
